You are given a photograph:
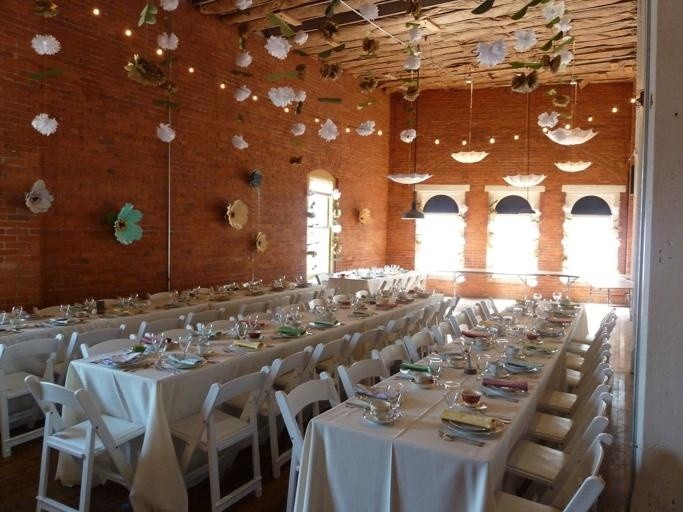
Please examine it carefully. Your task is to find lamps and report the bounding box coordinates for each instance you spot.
[553,83,594,172]
[543,46,599,148]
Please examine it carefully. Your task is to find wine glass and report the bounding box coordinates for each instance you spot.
[383,383,402,416]
[59,290,140,320]
[428,357,442,386]
[177,336,191,358]
[160,273,307,302]
[455,290,561,379]
[194,299,333,349]
[440,381,460,410]
[153,337,167,359]
[0,302,30,332]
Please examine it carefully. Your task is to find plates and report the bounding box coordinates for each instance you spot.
[411,381,434,389]
[439,415,504,437]
[459,400,486,412]
[362,412,396,425]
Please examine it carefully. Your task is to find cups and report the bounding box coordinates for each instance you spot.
[413,372,431,384]
[462,390,481,407]
[368,399,389,420]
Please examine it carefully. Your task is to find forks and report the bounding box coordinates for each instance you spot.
[438,430,484,447]
